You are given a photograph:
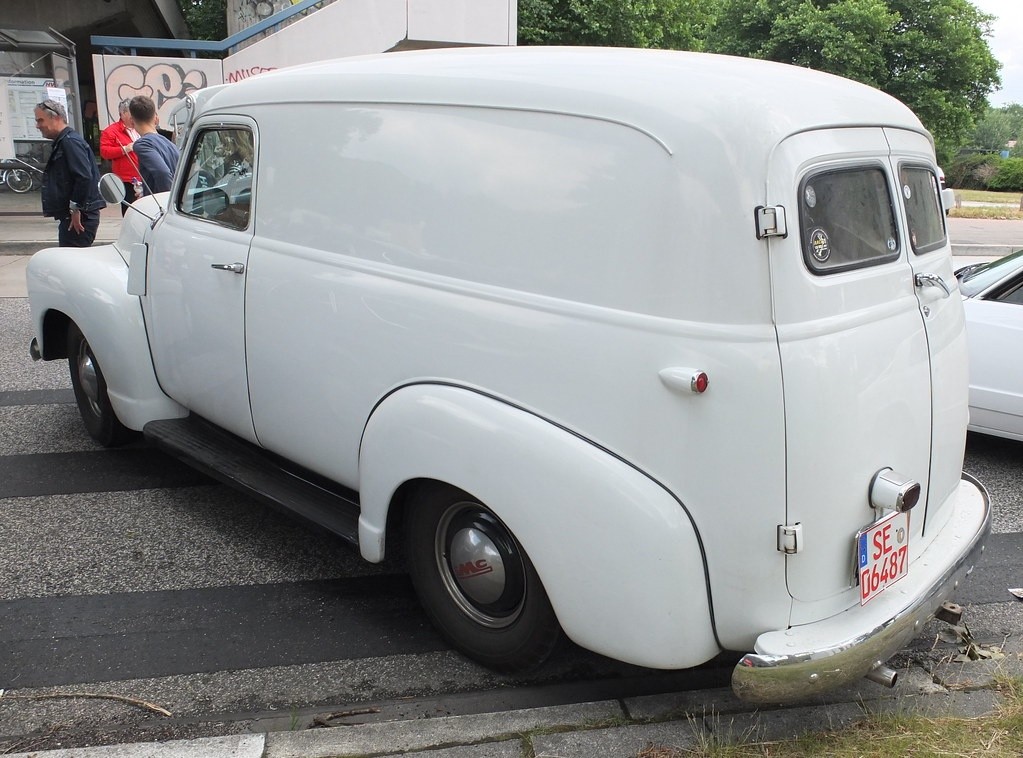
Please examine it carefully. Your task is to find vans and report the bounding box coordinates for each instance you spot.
[23,46,998,713]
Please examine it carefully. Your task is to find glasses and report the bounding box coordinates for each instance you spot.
[38,102,60,115]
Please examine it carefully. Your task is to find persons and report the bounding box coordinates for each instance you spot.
[34,100,108,246]
[100,99,141,217]
[129,94,181,197]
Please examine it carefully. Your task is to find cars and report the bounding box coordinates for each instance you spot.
[960,248,1023,444]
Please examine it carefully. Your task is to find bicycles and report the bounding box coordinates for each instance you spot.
[0,162,35,194]
